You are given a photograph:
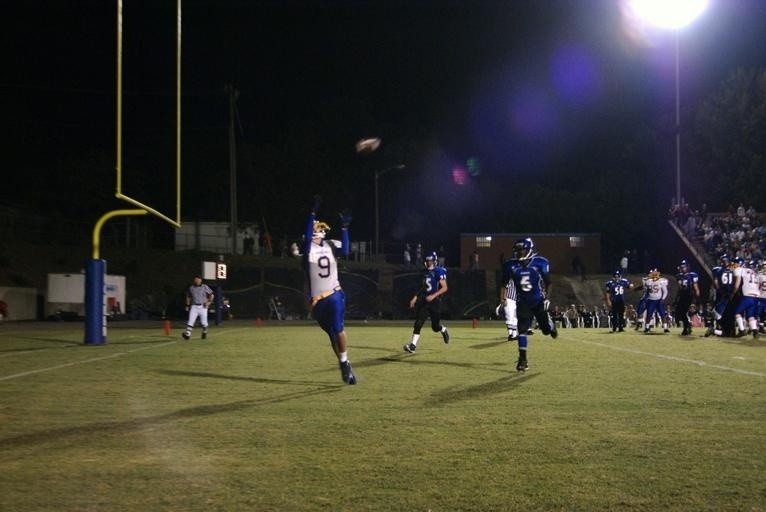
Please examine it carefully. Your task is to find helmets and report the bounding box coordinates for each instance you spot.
[311,220,330,238]
[733,257,745,267]
[720,254,730,266]
[424,252,437,269]
[650,268,660,279]
[680,259,689,272]
[749,261,759,268]
[513,238,535,260]
[613,271,622,279]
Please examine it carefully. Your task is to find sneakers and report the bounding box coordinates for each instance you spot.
[182,333,189,340]
[508,335,518,340]
[342,364,356,385]
[404,344,415,353]
[681,329,693,336]
[736,330,746,337]
[751,331,759,337]
[551,321,557,338]
[202,332,207,338]
[443,330,449,344]
[516,360,529,371]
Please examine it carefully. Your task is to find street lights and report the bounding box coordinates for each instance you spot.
[357,138,383,255]
[623,0,722,207]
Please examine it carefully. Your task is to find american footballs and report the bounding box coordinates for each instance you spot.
[355,138,382,153]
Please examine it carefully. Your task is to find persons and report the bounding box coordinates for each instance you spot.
[689,304,701,326]
[732,257,760,340]
[471,249,481,272]
[498,237,558,371]
[572,255,581,278]
[501,278,522,341]
[714,253,730,293]
[748,259,761,336]
[674,257,699,336]
[110,302,122,315]
[634,275,661,331]
[627,304,637,320]
[604,271,635,333]
[303,192,358,389]
[758,261,766,335]
[437,246,446,268]
[645,269,672,333]
[620,255,629,273]
[666,305,675,326]
[578,265,587,282]
[403,242,412,265]
[182,275,214,342]
[267,295,282,321]
[413,244,425,267]
[581,305,592,329]
[402,250,449,354]
[669,197,766,259]
[567,304,579,328]
[713,258,736,321]
[551,304,565,327]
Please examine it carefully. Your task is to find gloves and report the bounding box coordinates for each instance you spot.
[338,209,352,226]
[310,193,325,213]
[543,299,550,311]
